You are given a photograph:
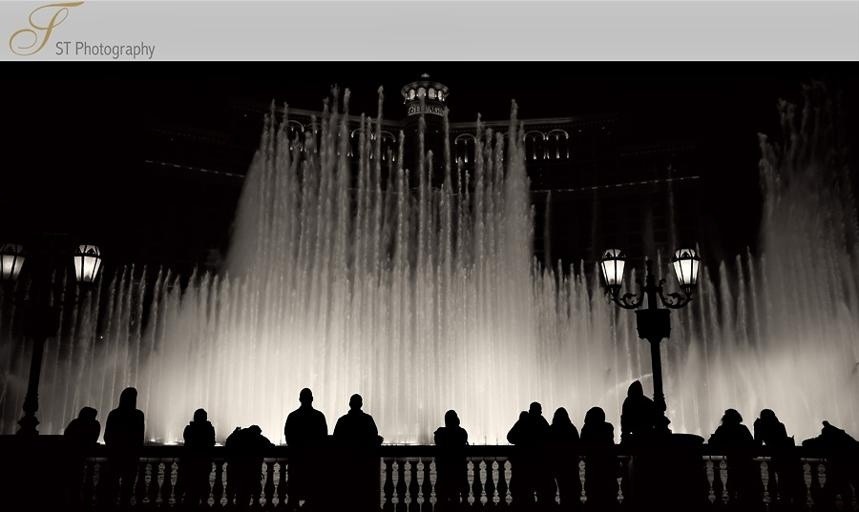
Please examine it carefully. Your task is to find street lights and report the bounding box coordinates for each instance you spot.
[0,211,112,435]
[596,232,702,438]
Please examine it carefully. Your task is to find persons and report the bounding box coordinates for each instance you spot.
[330,393,384,445]
[506,380,657,512]
[708,407,858,512]
[221,423,275,508]
[101,386,145,511]
[284,388,327,501]
[433,408,467,503]
[63,406,101,441]
[182,407,215,506]
